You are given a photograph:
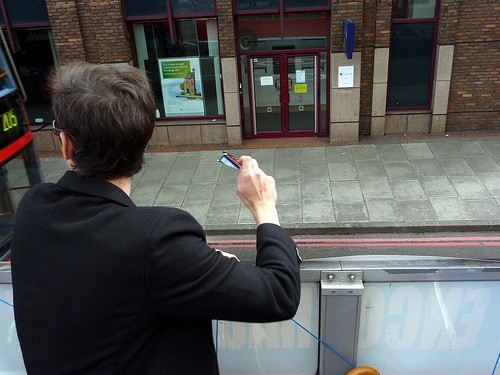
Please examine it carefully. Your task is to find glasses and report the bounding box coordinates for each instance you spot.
[51,119,76,142]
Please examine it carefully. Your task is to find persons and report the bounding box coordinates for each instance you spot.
[9,62,303,375]
